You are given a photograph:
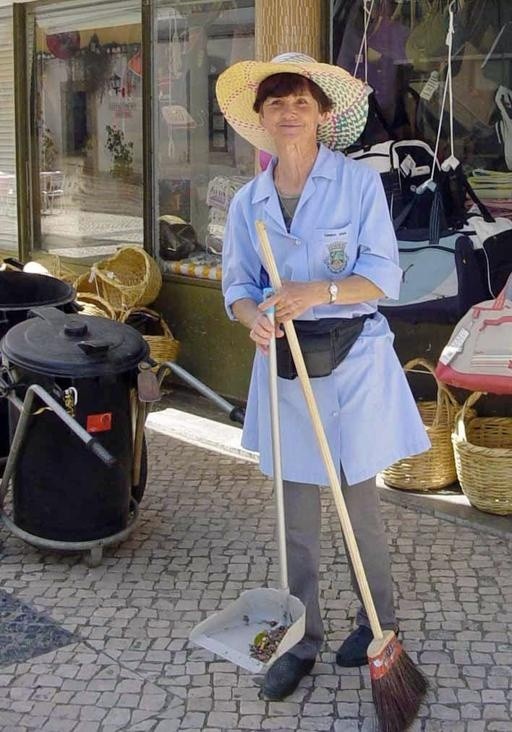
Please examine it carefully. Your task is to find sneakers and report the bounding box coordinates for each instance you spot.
[263,653,315,700]
[336,626,399,667]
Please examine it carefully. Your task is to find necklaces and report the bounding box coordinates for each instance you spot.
[274,162,294,221]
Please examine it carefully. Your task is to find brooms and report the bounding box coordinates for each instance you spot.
[256,220,429,732]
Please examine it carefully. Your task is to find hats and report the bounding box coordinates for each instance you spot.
[214,52,374,156]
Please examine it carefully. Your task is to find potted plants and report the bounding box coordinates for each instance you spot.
[104,124,136,182]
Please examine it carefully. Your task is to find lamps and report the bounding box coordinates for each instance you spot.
[109,72,125,96]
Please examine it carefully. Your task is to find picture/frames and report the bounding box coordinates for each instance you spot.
[206,72,231,153]
[156,178,193,225]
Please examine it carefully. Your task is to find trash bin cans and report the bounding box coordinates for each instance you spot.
[0,306,157,561]
[0,273,76,487]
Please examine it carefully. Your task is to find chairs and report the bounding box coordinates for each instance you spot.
[34,170,68,218]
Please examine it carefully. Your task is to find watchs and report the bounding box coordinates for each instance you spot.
[329,280,337,304]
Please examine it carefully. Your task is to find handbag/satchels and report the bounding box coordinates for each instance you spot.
[276,313,373,379]
[434,270,512,391]
[347,139,512,323]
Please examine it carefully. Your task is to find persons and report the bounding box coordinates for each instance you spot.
[211,49,435,703]
